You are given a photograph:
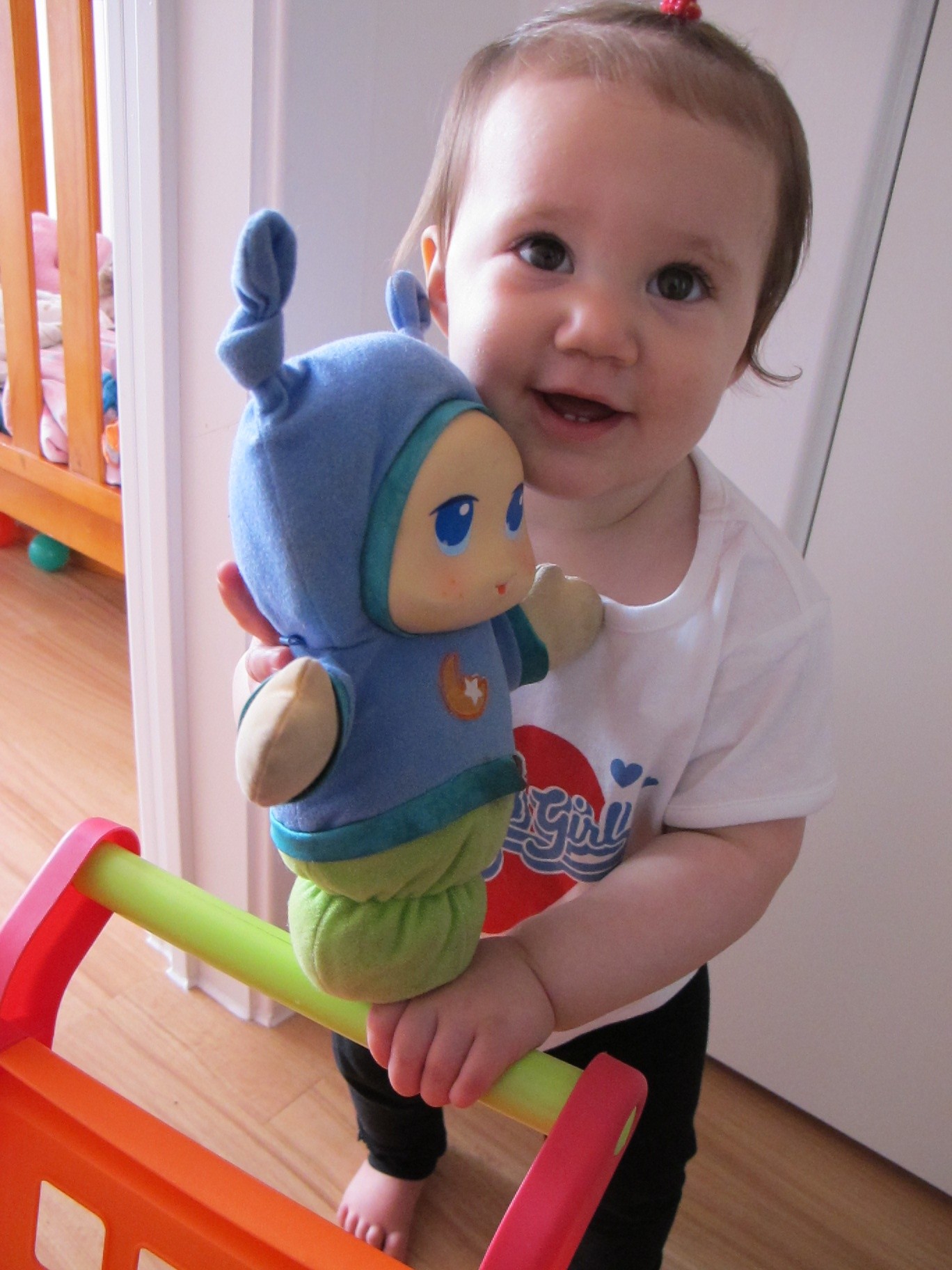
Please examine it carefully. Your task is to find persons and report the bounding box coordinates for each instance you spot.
[213,2,840,1268]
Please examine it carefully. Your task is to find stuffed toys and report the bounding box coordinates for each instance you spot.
[208,206,613,1003]
